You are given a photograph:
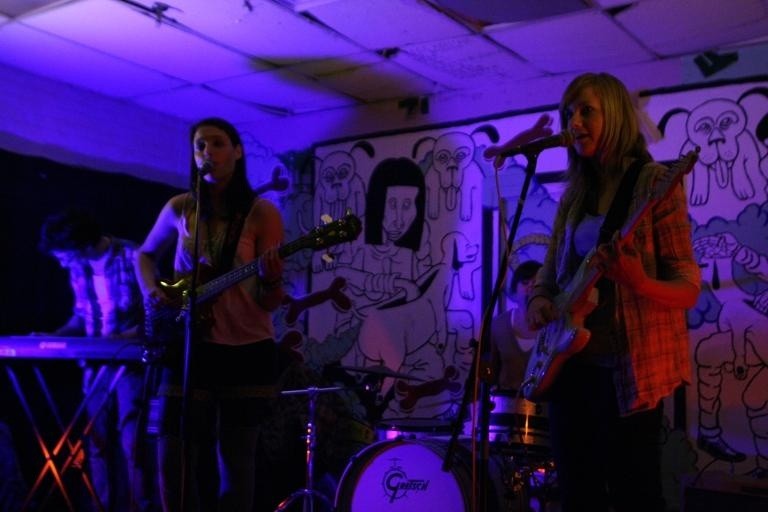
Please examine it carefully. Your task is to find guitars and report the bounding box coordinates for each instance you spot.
[523,147,701,399]
[146,214,361,358]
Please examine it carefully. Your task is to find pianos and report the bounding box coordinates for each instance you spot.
[0,335,146,361]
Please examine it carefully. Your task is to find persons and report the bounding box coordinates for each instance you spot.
[521,73,699,509]
[29,209,144,511]
[481,257,541,395]
[130,119,286,512]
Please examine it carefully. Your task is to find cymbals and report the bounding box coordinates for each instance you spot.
[340,365,425,382]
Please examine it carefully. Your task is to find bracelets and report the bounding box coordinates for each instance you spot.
[259,276,286,294]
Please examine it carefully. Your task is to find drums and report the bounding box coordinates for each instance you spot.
[369,418,455,440]
[275,488,333,510]
[460,386,553,447]
[334,438,513,512]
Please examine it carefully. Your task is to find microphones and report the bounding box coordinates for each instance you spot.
[502,129,575,154]
[198,162,213,178]
